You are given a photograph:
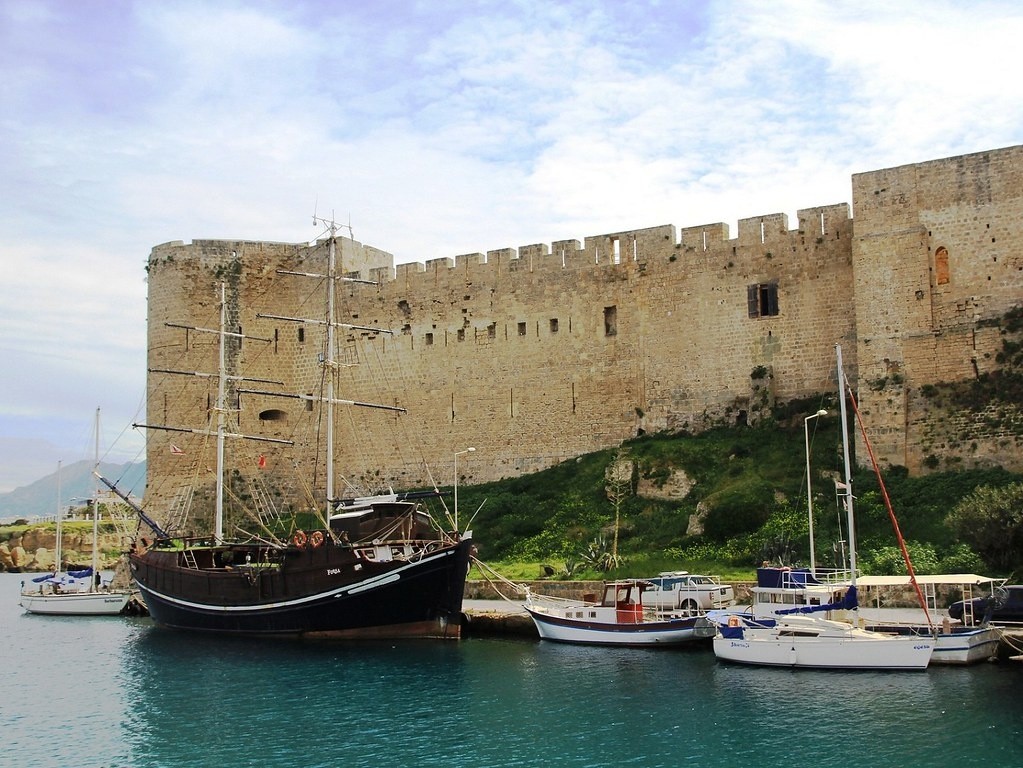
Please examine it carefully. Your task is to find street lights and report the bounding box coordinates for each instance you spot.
[805,409,828,579]
[453,446,477,540]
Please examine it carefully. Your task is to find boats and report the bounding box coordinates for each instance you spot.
[522,347,1023,672]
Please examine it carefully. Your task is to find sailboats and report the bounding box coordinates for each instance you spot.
[91,196,473,640]
[17,404,133,616]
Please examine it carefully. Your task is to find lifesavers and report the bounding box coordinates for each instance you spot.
[310,531,323,546]
[294,531,307,547]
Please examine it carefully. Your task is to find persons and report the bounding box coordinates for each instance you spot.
[95,572,101,593]
